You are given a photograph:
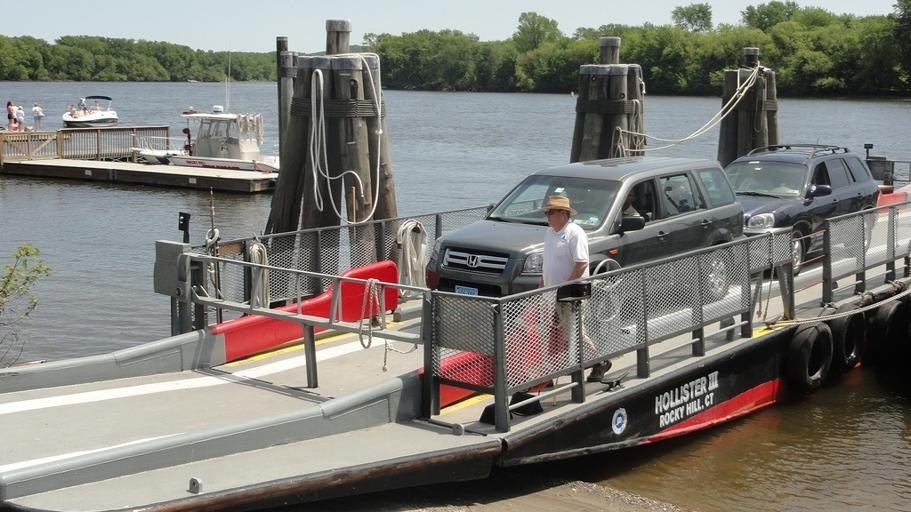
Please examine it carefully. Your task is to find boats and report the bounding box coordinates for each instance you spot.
[131,105,279,173]
[62,95,118,128]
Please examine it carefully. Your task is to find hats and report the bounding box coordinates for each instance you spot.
[539,195,578,218]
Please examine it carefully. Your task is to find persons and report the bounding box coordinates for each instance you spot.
[69,104,78,118]
[94,101,101,111]
[737,166,774,192]
[587,186,640,221]
[538,194,613,382]
[15,105,26,132]
[78,97,90,110]
[11,118,19,132]
[31,102,44,132]
[6,101,15,132]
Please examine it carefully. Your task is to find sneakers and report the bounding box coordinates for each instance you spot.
[587,359,611,382]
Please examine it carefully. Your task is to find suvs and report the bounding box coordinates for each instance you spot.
[424,157,743,323]
[724,143,881,276]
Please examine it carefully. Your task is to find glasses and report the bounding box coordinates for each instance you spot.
[542,208,562,215]
